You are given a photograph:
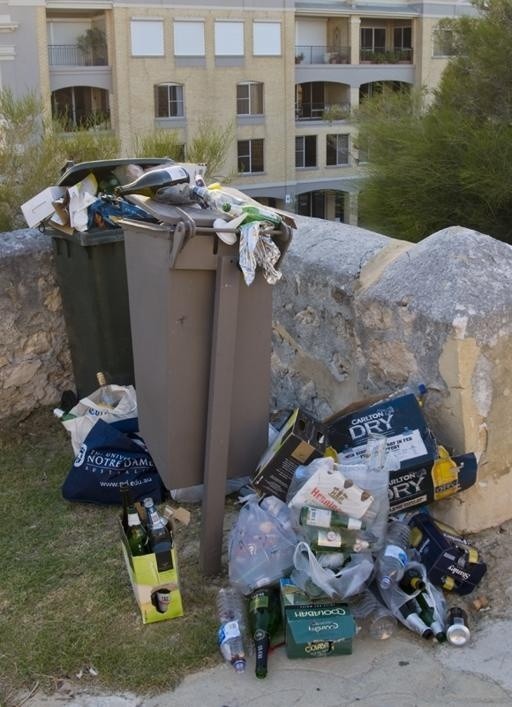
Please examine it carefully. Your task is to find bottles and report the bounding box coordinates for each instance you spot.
[96,372,116,406]
[115,166,282,223]
[122,497,447,679]
[53,408,77,421]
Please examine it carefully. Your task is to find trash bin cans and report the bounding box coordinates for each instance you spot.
[115,192,294,490]
[43,157,175,403]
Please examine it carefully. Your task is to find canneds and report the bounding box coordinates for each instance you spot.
[446,607,471,647]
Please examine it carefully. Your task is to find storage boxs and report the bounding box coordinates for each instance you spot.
[117,508,191,625]
[253,407,328,493]
[405,514,487,597]
[20,185,65,227]
[280,578,354,657]
[325,394,477,515]
